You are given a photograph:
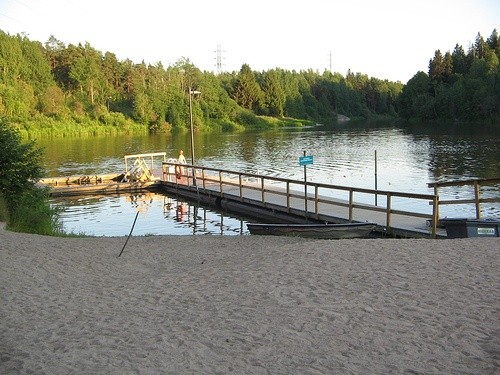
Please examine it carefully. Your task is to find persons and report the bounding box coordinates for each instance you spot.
[177,150,187,176]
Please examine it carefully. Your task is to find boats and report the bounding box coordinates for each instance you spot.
[27,152,178,194]
[246,221,378,239]
